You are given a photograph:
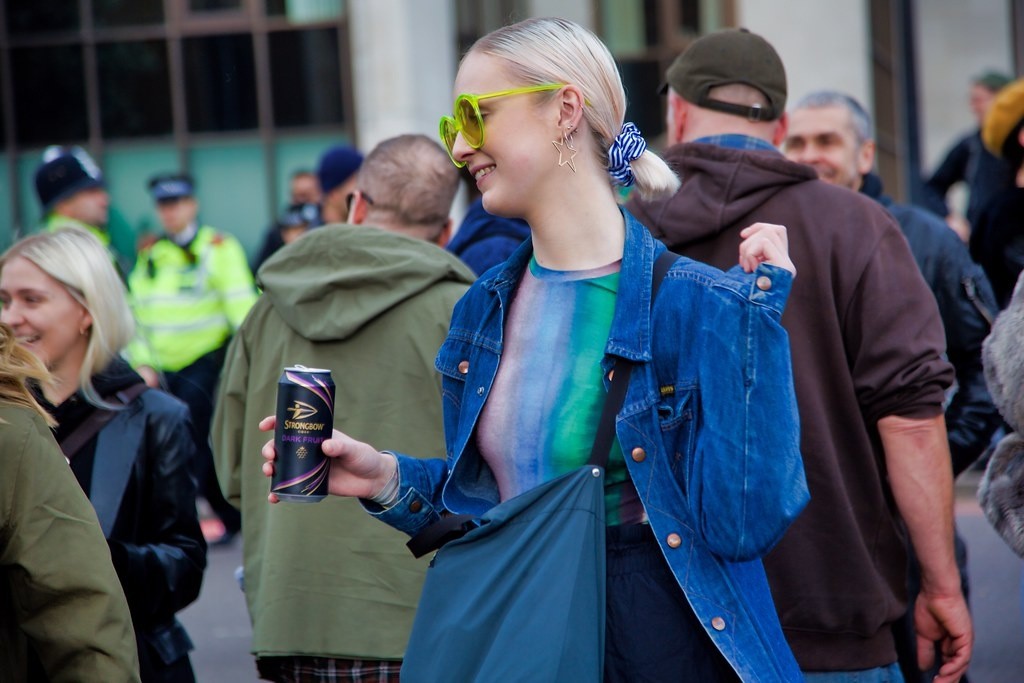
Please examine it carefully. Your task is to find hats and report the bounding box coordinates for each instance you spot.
[983,78,1024,164]
[656,28,787,121]
[973,72,1010,91]
[318,150,362,193]
[148,175,192,199]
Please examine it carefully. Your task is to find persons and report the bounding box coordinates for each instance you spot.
[615,27,1024,683]
[0,134,532,683]
[258,16,811,683]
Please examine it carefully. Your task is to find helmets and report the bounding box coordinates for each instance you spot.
[35,146,101,202]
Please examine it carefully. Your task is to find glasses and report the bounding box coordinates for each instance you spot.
[439,82,594,169]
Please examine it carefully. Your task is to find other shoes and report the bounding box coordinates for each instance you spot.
[211,533,236,543]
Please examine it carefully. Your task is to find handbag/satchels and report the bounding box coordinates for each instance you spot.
[400,251,692,683]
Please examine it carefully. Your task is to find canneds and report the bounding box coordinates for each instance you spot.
[268,365,335,503]
